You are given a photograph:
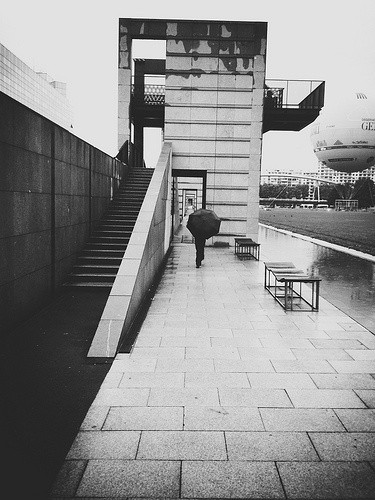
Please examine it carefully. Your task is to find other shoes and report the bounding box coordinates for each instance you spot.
[199,261,201,265]
[196,264,199,268]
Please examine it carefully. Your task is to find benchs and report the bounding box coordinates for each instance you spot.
[264,261,322,311]
[234,237,261,261]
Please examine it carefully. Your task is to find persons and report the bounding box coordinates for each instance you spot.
[194,237,206,268]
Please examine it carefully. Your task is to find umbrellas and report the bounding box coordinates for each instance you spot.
[186,208,221,240]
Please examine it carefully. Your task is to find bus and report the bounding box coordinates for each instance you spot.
[300,203,329,208]
